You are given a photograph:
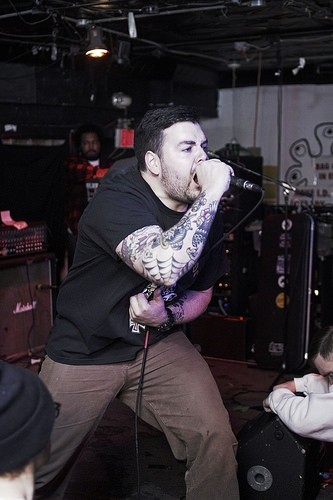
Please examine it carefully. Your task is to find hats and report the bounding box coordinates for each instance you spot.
[0,361,54,472]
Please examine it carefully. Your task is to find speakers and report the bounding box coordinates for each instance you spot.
[0,252,54,362]
[234,409,321,500]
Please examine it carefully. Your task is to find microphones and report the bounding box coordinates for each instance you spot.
[35,284,57,290]
[230,175,264,193]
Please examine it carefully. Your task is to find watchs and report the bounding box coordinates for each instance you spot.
[158,308,174,332]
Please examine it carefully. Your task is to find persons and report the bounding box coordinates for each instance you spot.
[60,124,116,281]
[34,105,240,500]
[262,326,333,442]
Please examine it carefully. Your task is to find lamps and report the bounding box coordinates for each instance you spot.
[85,26,108,58]
